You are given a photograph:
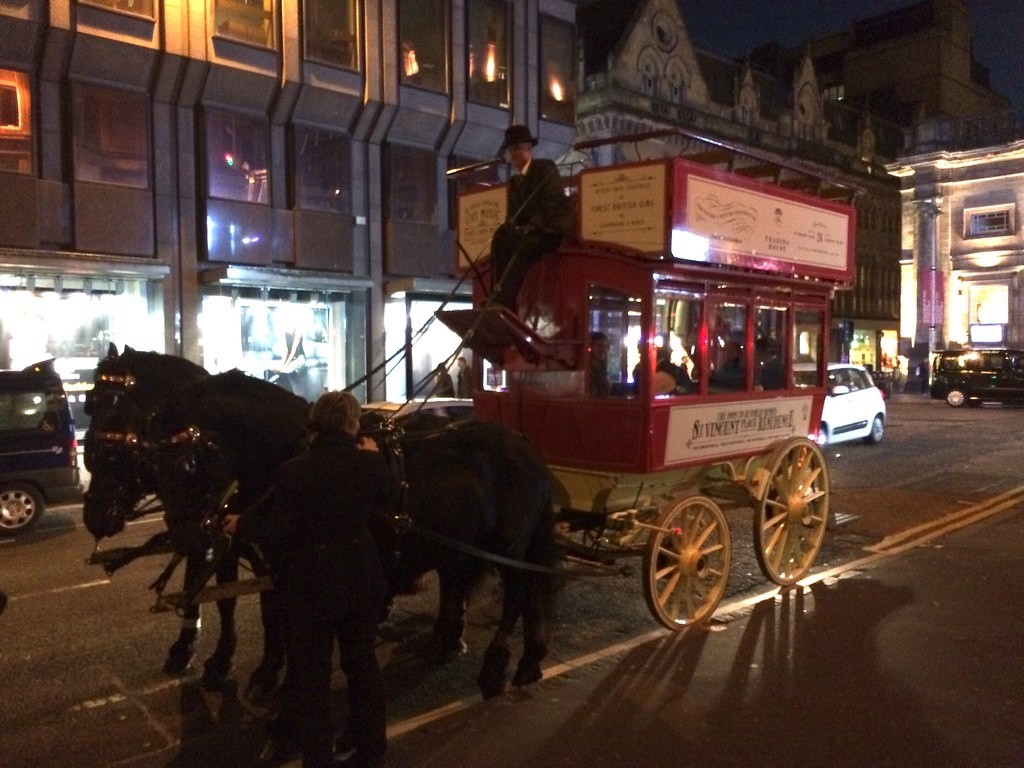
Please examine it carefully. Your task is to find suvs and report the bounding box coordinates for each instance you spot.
[0,358,79,537]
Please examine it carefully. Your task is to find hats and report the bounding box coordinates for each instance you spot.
[500,124,539,148]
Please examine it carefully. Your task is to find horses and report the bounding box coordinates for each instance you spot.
[80,340,569,768]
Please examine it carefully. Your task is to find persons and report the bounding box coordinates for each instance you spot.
[882,353,888,370]
[682,356,688,369]
[482,124,574,316]
[277,327,307,373]
[691,335,783,393]
[222,392,402,768]
[435,363,455,397]
[590,333,609,395]
[457,358,473,398]
[631,332,695,394]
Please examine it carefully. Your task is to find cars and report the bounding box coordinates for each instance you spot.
[793,362,887,454]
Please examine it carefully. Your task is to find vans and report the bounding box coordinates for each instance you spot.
[932,349,1024,408]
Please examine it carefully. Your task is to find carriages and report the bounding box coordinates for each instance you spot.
[79,128,857,768]
